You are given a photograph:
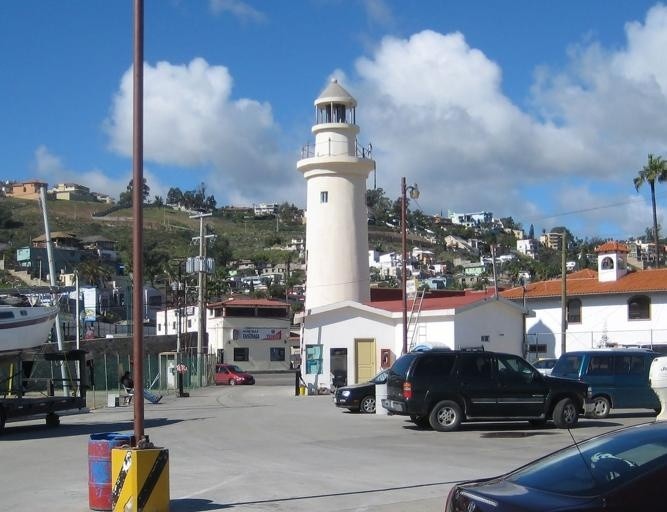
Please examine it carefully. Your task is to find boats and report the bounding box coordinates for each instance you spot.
[0,295,59,352]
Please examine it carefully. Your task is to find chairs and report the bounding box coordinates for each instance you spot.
[122,383,134,404]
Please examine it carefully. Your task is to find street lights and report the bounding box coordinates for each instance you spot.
[401,176,420,353]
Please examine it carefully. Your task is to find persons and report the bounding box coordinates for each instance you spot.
[121,371,163,403]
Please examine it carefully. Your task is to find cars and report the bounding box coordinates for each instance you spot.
[335,368,392,414]
[444,419,666,511]
[208,363,255,386]
[387,348,664,431]
